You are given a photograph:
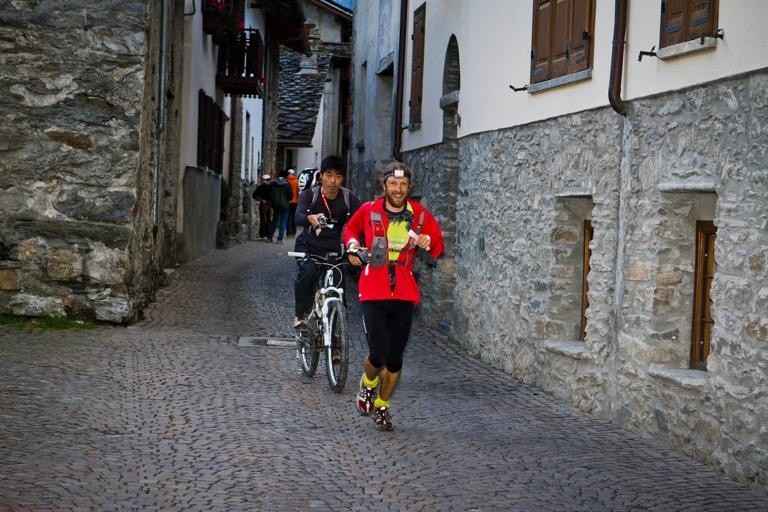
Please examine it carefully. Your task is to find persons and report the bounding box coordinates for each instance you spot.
[342,164,445,433]
[294,154,366,362]
[251,169,298,246]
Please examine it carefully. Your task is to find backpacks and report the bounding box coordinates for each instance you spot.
[271,180,289,208]
[296,168,352,216]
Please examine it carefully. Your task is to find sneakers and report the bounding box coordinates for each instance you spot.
[370,406,392,431]
[293,316,309,327]
[355,372,380,416]
[331,348,342,363]
[257,237,284,244]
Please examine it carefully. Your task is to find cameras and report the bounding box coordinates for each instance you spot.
[318,216,339,226]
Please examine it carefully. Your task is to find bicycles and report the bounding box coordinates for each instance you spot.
[287,250,351,393]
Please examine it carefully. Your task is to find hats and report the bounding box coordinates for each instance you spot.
[288,169,295,175]
[262,174,270,181]
